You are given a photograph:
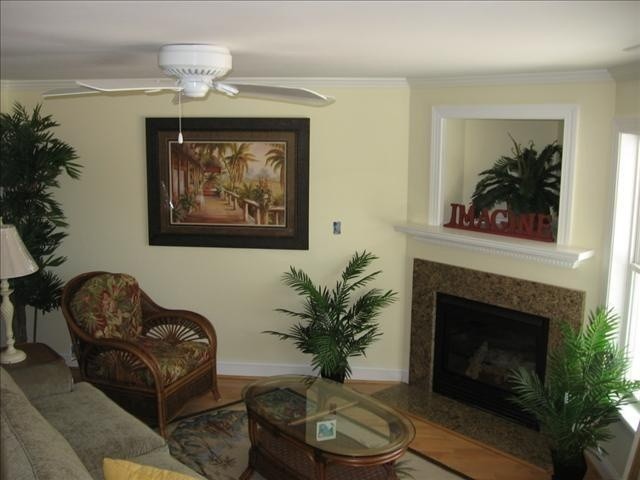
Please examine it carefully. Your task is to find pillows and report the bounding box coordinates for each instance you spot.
[102,456,203,480]
[69,273,143,357]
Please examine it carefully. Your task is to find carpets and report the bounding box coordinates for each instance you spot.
[151,387,476,479]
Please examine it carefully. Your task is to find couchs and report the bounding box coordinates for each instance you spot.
[0,360,208,480]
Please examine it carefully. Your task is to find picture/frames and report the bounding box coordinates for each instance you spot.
[144,117,310,250]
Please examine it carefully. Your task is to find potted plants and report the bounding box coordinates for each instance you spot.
[259,249,401,384]
[468,132,563,231]
[504,303,640,480]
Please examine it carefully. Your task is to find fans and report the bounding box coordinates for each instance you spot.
[42,44,329,145]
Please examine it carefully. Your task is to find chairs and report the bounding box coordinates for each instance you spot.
[61,271,223,440]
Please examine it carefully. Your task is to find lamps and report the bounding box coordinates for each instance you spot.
[0,216,40,365]
